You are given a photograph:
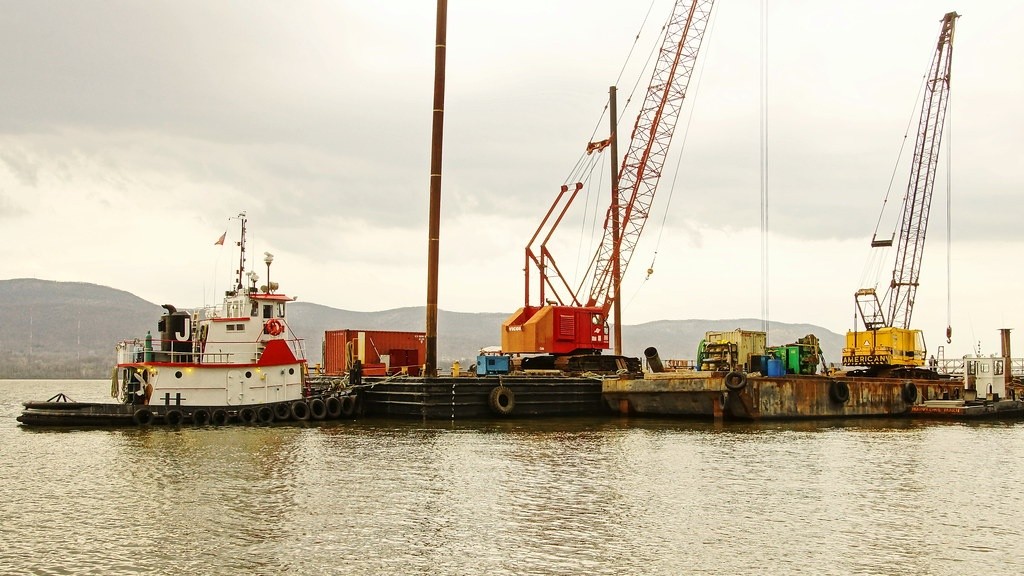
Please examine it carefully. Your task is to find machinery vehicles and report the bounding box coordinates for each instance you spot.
[839,10,964,381]
[497,1,720,374]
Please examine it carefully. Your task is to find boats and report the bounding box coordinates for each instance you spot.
[13,208,372,430]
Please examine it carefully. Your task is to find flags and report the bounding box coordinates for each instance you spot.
[215,232,227,245]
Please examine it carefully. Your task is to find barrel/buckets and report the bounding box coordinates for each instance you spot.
[767,359,782,377]
[760,355,770,373]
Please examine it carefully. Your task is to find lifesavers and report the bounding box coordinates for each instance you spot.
[266,318,282,336]
